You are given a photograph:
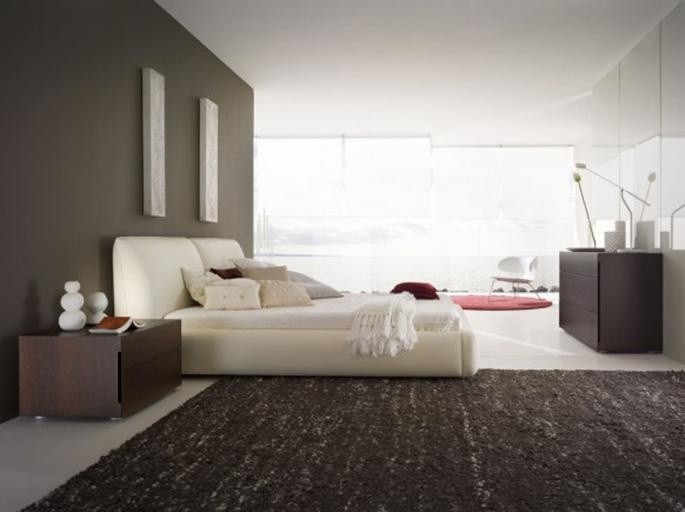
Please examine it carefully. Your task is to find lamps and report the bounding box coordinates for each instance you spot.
[571,160,652,252]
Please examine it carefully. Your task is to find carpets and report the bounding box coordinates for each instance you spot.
[11,367,685,512]
[449,293,554,312]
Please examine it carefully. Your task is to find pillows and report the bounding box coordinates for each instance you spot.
[179,266,225,307]
[204,277,262,312]
[210,258,343,301]
[260,280,314,306]
[390,281,438,301]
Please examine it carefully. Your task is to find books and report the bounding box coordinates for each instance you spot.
[86,315,146,334]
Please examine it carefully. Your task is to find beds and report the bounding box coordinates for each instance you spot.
[112,234,478,380]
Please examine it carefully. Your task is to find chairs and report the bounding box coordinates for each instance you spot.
[486,254,544,304]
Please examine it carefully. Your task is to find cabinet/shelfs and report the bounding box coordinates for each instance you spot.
[557,250,665,357]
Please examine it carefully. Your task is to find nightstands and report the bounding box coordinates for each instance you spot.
[16,317,184,423]
